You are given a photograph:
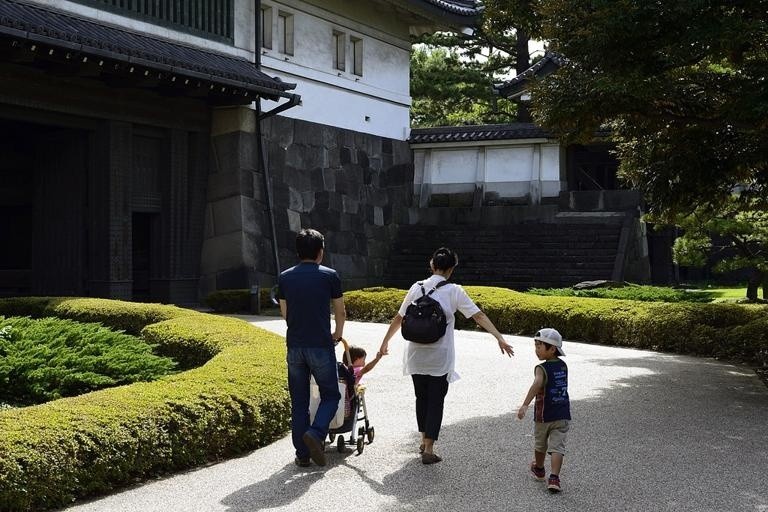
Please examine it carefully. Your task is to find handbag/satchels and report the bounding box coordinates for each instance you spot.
[309,364,347,429]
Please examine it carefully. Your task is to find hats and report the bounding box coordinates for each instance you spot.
[534,328,565,356]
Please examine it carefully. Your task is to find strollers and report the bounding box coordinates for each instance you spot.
[320,337,375,452]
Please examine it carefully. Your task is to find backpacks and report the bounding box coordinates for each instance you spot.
[401,281,452,343]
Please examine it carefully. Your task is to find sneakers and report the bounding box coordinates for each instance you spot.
[548,479,563,493]
[531,461,546,482]
[419,444,442,464]
[295,430,327,467]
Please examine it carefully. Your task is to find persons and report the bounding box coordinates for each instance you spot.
[379,247,514,465]
[517,328,571,491]
[277,228,347,467]
[339,346,382,419]
